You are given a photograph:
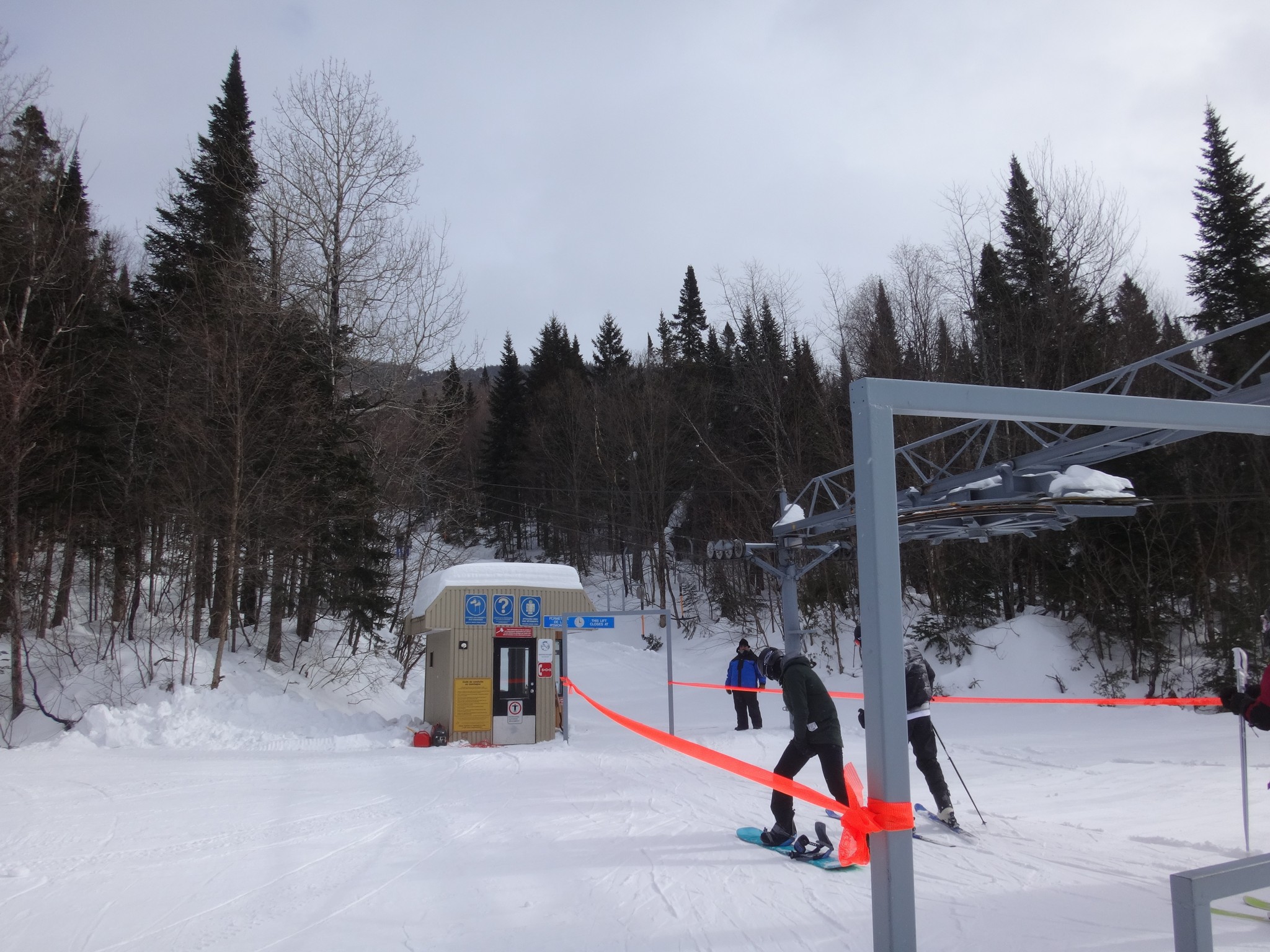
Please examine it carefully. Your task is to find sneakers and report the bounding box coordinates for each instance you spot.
[753,726,761,730]
[735,726,749,731]
[761,820,795,844]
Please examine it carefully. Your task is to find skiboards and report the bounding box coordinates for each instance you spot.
[821,802,981,849]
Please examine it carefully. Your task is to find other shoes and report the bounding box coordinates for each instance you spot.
[933,789,958,828]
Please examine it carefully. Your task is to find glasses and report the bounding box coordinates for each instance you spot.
[740,644,748,647]
[854,636,862,647]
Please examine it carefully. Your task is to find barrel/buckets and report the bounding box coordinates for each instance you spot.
[405,726,431,748]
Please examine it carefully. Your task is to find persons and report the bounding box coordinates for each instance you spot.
[756,647,850,846]
[1219,610,1270,731]
[725,638,766,731]
[854,622,955,824]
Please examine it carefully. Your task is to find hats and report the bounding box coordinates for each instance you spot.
[736,638,750,653]
[854,623,861,642]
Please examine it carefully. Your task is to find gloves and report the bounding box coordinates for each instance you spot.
[793,737,807,753]
[858,708,866,729]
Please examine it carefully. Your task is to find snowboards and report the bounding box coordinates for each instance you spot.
[738,825,851,871]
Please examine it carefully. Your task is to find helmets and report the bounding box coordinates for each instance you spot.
[757,647,785,681]
